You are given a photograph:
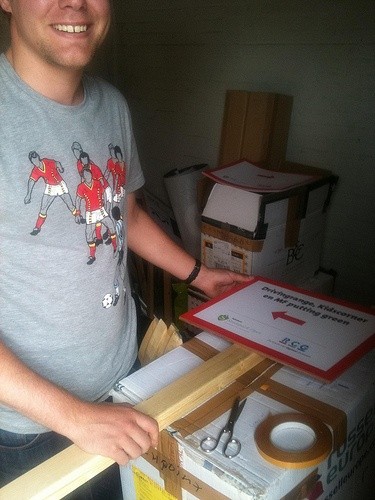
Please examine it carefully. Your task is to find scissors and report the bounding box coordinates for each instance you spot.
[200,394,247,458]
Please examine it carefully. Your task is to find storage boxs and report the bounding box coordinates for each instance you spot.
[109,161,375,500]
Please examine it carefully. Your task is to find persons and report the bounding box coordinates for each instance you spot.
[1,1,253,499]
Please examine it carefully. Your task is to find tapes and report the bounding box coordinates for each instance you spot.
[254,414,332,469]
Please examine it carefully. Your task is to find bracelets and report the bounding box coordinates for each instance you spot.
[183,259,201,284]
